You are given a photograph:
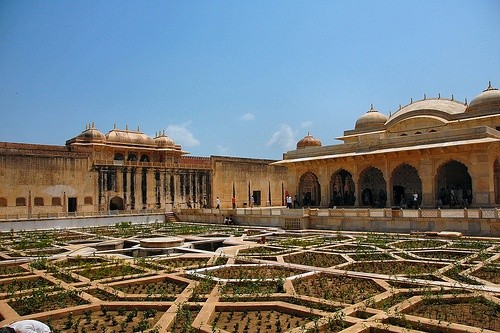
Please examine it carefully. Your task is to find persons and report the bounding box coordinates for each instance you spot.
[224,217,236,225]
[251,195,255,208]
[0,320,52,333]
[302,192,310,206]
[202,197,207,208]
[378,189,386,207]
[187,198,192,209]
[336,191,355,206]
[400,192,420,210]
[435,197,442,209]
[286,194,300,209]
[363,188,370,206]
[440,184,472,208]
[216,197,220,209]
[232,195,236,209]
